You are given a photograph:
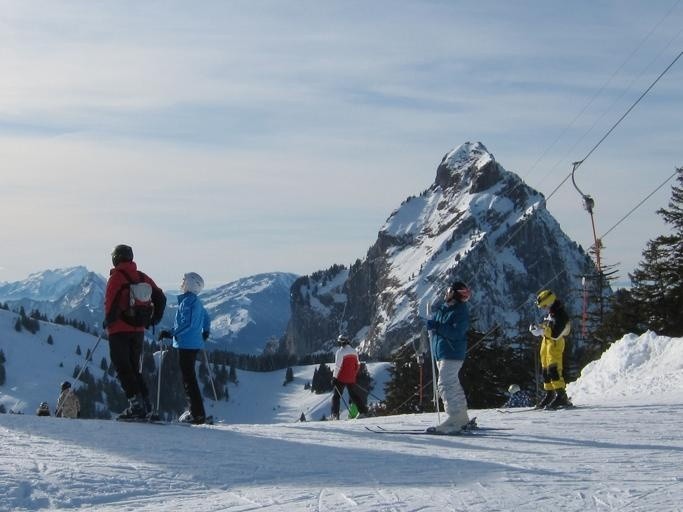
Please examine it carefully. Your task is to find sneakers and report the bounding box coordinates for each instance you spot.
[179,410,205,423]
[327,415,339,421]
[356,413,368,418]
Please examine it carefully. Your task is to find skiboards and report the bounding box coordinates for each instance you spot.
[113,417,228,427]
[365,425,515,437]
[497,406,598,413]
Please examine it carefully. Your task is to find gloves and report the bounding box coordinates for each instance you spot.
[158,331,173,341]
[528,323,544,337]
[427,320,437,330]
[330,378,337,387]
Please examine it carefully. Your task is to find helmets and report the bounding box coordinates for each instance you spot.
[182,273,204,295]
[112,245,133,266]
[537,291,555,308]
[61,381,71,389]
[450,282,470,303]
[337,334,349,343]
[508,384,520,394]
[40,402,49,409]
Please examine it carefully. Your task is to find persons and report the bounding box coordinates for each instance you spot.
[35,401,51,416]
[322,334,371,421]
[527,288,572,410]
[53,380,80,418]
[157,271,211,423]
[99,245,167,423]
[500,383,533,409]
[422,281,472,435]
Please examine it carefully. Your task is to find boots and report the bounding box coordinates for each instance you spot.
[537,390,570,410]
[117,394,152,418]
[435,408,468,433]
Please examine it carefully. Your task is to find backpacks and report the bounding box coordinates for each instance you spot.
[116,268,156,327]
